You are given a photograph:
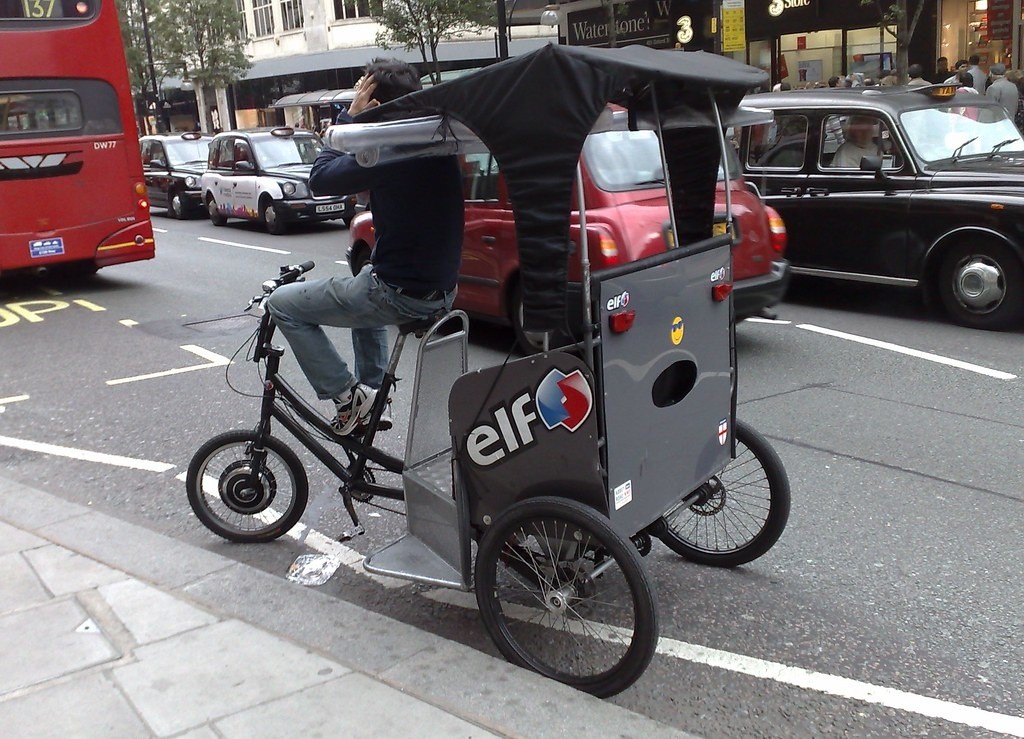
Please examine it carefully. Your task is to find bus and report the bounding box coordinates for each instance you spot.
[0,0,155,290]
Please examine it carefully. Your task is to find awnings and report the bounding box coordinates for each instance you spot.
[132,37,559,96]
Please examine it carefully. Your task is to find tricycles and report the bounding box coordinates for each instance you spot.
[185,43,800,699]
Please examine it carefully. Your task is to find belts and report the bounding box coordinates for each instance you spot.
[383,282,456,301]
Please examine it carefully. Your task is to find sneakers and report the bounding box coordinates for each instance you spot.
[330,381,379,436]
[359,396,393,431]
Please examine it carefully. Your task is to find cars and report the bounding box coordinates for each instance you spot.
[345,100,795,358]
[139,131,216,221]
[724,80,1024,333]
[200,124,359,236]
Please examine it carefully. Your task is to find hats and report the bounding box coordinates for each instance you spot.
[990,63,1006,75]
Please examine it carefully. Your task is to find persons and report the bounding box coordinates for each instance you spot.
[766,56,1024,148]
[267,58,465,436]
[830,114,886,168]
